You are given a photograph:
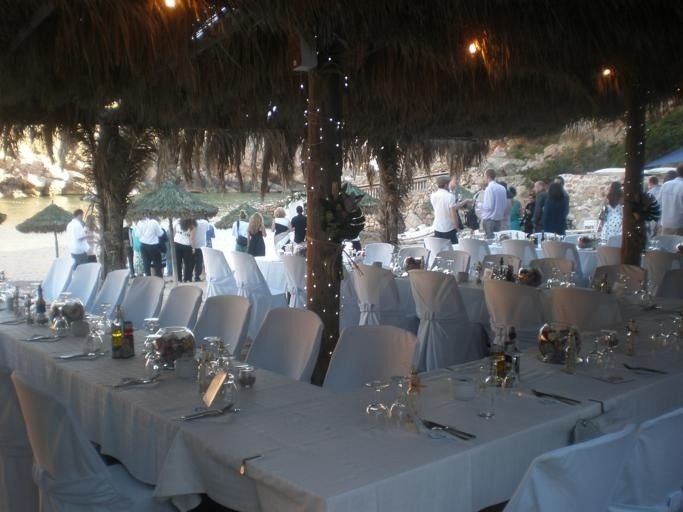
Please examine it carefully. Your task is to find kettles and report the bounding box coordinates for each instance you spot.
[535,321,582,364]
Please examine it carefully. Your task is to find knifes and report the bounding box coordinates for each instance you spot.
[55,346,109,361]
[17,332,68,343]
[111,372,160,388]
[177,403,241,425]
[422,417,474,442]
[530,388,580,408]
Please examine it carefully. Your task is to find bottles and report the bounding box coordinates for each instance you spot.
[623,318,637,357]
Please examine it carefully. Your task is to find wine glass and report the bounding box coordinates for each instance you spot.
[646,239,663,253]
[82,302,111,352]
[447,228,565,247]
[0,268,82,334]
[475,348,522,417]
[585,332,618,372]
[361,378,414,441]
[649,311,682,357]
[349,247,656,305]
[141,315,165,377]
[198,335,238,402]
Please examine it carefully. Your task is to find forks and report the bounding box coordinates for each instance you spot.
[623,362,668,375]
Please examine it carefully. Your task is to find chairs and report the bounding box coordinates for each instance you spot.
[0,222,683,511]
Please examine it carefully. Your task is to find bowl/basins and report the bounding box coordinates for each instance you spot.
[447,374,478,402]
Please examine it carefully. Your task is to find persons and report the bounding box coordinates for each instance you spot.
[350,218,361,256]
[598,166,682,245]
[271,203,307,255]
[231,208,267,256]
[578,236,592,247]
[430,168,569,244]
[66,209,101,268]
[124,216,215,282]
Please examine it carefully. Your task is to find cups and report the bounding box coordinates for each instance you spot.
[111,304,133,358]
[236,366,254,387]
